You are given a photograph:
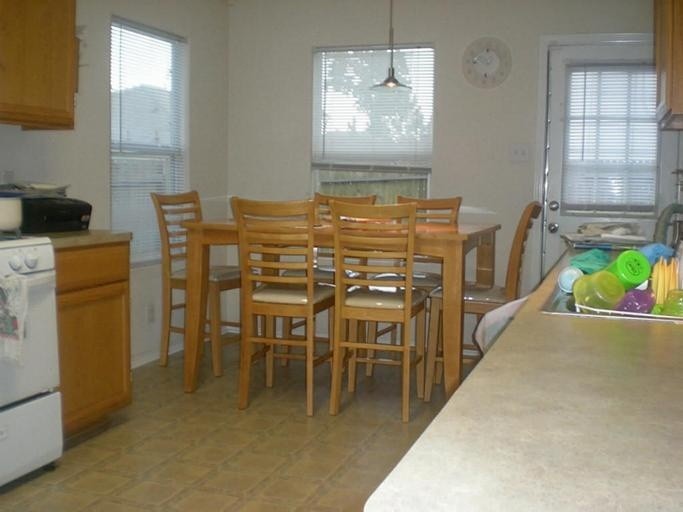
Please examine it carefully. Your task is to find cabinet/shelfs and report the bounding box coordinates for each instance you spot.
[653,0,683,131]
[24,230,133,448]
[0,0,75,131]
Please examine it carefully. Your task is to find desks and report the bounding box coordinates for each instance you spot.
[363,226,683,512]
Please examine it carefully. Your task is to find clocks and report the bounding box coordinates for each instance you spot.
[460,37,513,89]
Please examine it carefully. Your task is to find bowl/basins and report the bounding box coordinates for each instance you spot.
[573,271,626,315]
[558,267,584,293]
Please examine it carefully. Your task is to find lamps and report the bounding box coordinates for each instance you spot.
[368,1,412,95]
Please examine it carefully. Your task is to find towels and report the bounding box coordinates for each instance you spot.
[1,274,29,364]
[638,242,675,267]
[570,249,611,274]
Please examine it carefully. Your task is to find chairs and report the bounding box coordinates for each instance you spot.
[328,198,428,424]
[361,196,462,380]
[281,192,376,374]
[424,202,544,405]
[229,196,346,417]
[149,189,261,378]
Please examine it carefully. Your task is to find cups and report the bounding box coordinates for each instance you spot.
[659,288,683,318]
[605,250,651,292]
[616,290,656,316]
[640,244,668,265]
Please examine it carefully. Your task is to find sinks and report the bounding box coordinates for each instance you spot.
[539,280,682,322]
